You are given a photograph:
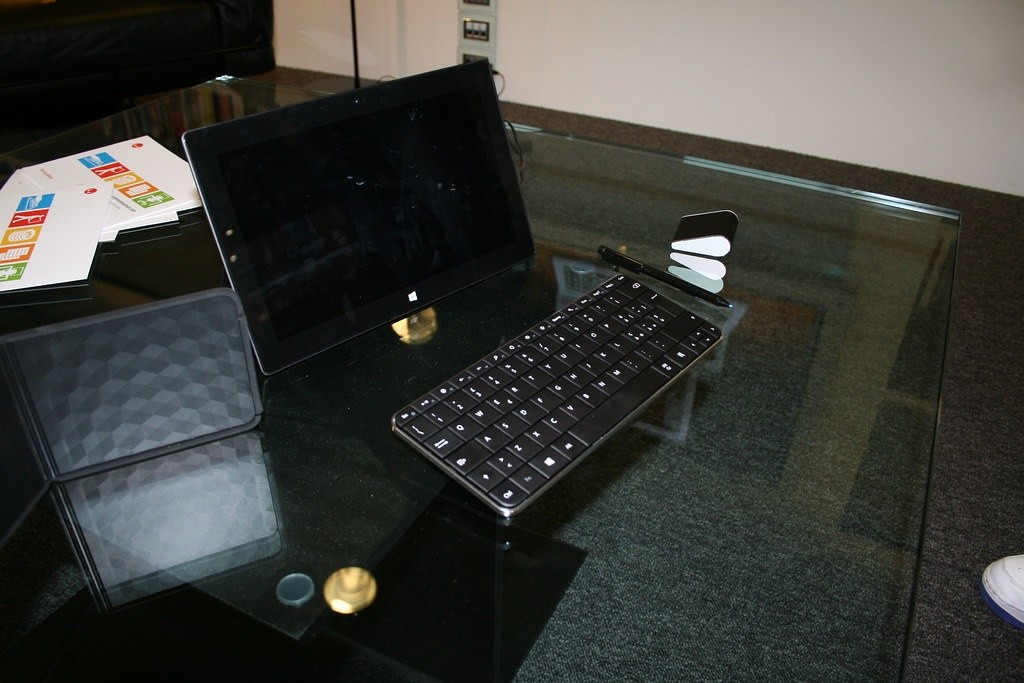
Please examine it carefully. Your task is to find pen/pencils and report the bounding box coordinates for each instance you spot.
[600,242,734,310]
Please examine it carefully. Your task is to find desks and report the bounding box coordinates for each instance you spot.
[0,71,1024,683]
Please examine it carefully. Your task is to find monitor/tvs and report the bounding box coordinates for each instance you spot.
[179,60,532,375]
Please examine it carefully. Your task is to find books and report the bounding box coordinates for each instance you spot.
[0,135,204,293]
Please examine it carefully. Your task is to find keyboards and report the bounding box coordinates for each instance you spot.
[391,273,723,516]
